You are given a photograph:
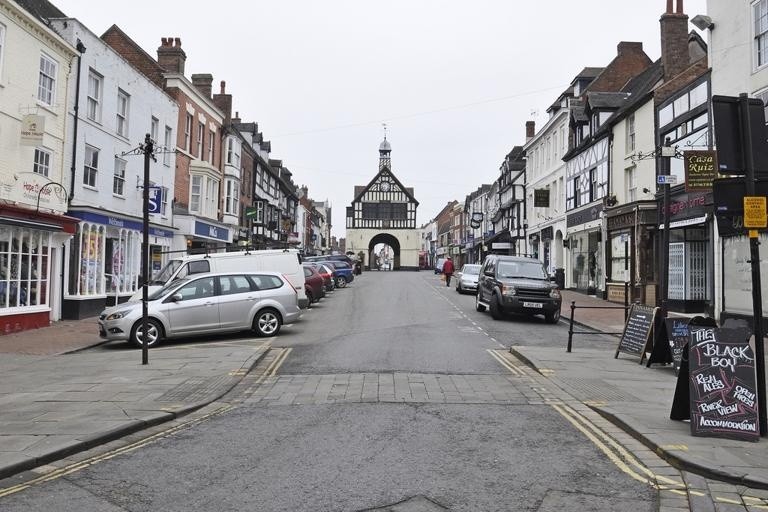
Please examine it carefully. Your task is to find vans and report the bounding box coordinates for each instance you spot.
[126,247,310,310]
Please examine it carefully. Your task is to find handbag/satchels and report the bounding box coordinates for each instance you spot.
[440,273,447,281]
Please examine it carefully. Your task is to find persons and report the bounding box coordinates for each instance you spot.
[443,256,455,287]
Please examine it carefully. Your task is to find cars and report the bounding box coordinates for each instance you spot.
[435,258,448,274]
[455,263,482,294]
[300,254,355,303]
[97,270,302,351]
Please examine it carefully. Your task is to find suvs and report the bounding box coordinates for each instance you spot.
[475,254,563,324]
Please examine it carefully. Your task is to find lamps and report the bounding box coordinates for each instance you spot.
[690,15,716,33]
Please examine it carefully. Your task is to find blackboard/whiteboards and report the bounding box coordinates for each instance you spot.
[689,326,760,443]
[619,302,660,355]
[665,318,690,374]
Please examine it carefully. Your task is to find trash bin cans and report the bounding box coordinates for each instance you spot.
[555,269,565,290]
